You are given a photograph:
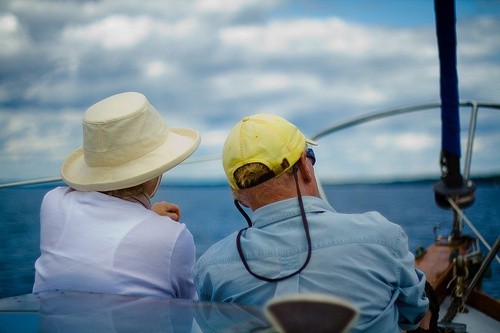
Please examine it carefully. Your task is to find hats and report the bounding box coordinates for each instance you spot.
[60,91,201,191]
[221,113,318,190]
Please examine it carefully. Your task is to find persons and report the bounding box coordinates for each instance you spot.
[193,111,453,333]
[33,91,200,302]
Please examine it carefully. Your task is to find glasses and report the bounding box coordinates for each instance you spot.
[294,149,316,168]
